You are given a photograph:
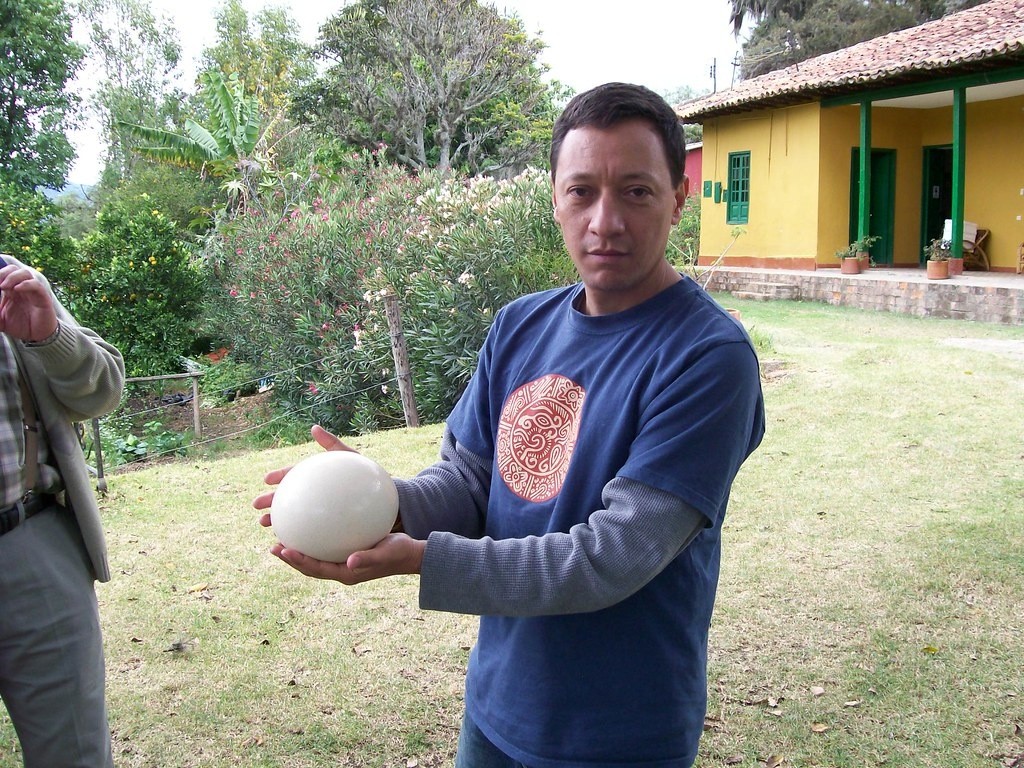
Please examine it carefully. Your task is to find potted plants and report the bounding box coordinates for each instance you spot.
[923,239,952,280]
[833,235,883,274]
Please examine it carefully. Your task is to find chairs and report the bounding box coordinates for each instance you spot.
[962,228,990,271]
[1016,241,1024,274]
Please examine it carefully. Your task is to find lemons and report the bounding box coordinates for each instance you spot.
[0,200,178,301]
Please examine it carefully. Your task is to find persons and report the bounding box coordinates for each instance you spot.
[0,254,126,768]
[254,83,766,767]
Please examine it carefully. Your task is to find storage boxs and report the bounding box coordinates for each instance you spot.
[941,219,977,253]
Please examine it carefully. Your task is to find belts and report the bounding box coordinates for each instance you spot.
[0,494,53,536]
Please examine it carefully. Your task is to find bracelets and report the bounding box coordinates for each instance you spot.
[21,323,60,347]
